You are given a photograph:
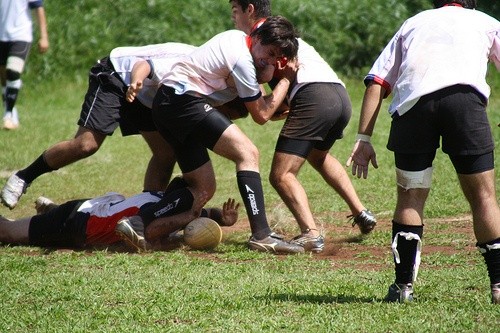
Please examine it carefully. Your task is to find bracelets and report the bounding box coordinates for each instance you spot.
[356,133,371,143]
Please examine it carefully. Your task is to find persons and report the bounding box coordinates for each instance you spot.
[0,0,50,131]
[0,43,250,210]
[154,16,304,254]
[229,0,377,251]
[347,0,500,305]
[0,176,241,253]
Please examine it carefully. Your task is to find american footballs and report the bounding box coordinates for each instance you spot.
[184,217,222,249]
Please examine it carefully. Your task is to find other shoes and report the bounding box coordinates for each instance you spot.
[247,231,305,255]
[1,107,19,130]
[34,197,55,213]
[0,169,32,210]
[289,235,324,254]
[114,216,153,253]
[346,209,377,234]
[383,281,413,302]
[490,283,499,303]
